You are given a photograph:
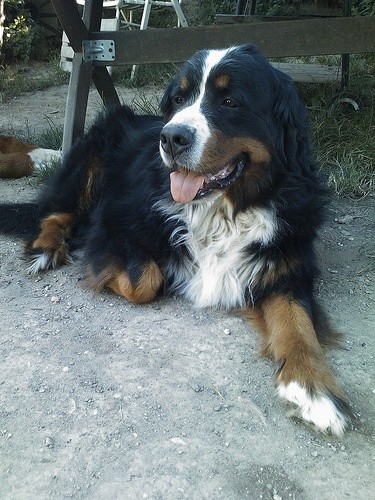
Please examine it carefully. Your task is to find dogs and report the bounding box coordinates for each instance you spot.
[0,41,358,438]
[0,135,64,181]
